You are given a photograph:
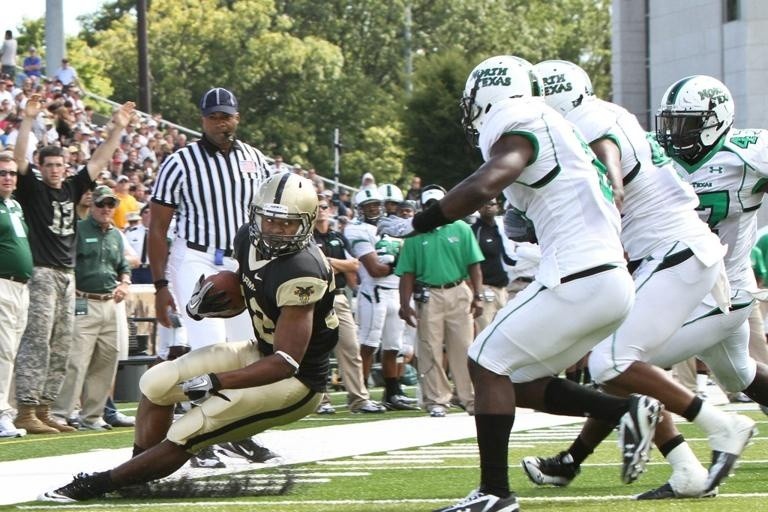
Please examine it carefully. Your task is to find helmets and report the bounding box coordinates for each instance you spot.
[356,184,447,208]
[532,60,593,114]
[249,173,318,257]
[656,75,734,161]
[460,56,540,146]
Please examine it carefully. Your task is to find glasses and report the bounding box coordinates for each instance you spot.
[485,201,497,205]
[94,200,117,208]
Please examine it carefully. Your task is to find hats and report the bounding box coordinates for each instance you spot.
[92,187,120,207]
[202,88,236,116]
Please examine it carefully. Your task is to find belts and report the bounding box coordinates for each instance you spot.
[0,274,27,283]
[517,277,532,282]
[187,243,235,257]
[424,280,464,289]
[76,292,113,299]
[335,289,346,295]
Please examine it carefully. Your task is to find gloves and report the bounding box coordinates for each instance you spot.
[176,374,214,404]
[186,273,232,321]
[504,209,537,243]
[375,215,414,240]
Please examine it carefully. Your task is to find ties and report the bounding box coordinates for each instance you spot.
[141,231,150,263]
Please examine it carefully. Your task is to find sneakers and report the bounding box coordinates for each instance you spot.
[317,395,446,417]
[41,472,110,502]
[0,406,135,437]
[191,438,280,468]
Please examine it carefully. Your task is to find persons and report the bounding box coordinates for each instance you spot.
[372,55,767,510]
[0,30,187,437]
[145,87,279,472]
[273,155,488,416]
[39,175,339,501]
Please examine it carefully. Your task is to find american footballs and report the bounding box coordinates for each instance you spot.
[205,271,246,318]
